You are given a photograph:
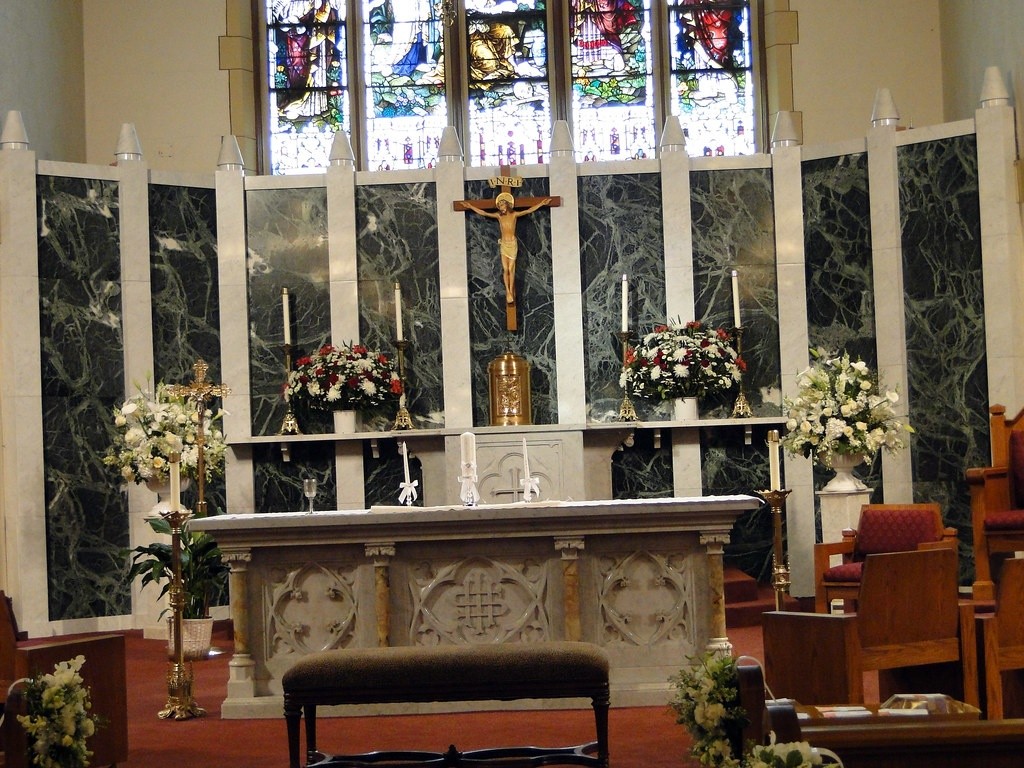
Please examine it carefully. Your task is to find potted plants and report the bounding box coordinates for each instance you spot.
[123,506,232,663]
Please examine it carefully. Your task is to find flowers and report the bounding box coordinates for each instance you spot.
[13,655,110,768]
[278,339,404,416]
[662,642,844,768]
[610,315,747,419]
[98,368,232,489]
[761,345,911,466]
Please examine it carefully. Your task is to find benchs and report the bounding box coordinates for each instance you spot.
[282,640,612,768]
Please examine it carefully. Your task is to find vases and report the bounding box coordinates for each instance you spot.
[144,474,191,517]
[334,410,363,433]
[820,453,867,492]
[676,397,699,421]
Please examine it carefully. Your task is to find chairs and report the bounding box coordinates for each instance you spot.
[737,402,1024,768]
[0,590,128,768]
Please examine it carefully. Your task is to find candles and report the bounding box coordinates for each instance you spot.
[168,449,181,512]
[522,437,529,479]
[282,287,291,345]
[402,441,410,484]
[767,430,780,491]
[622,273,628,333]
[394,282,403,342]
[731,270,741,329]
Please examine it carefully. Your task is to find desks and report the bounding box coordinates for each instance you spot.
[185,494,765,719]
